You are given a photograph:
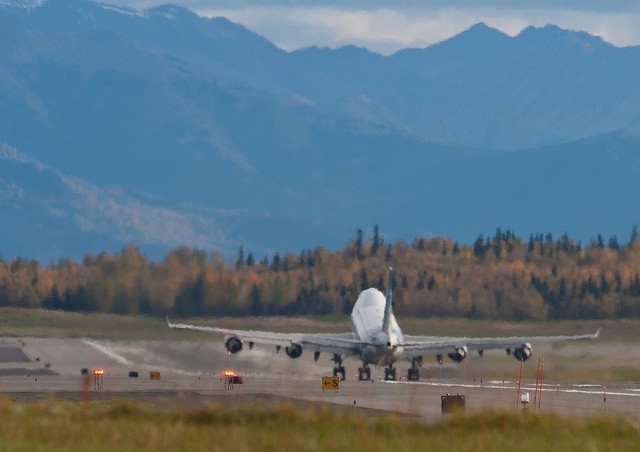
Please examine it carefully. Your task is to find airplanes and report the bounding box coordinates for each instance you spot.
[166,265,602,382]
